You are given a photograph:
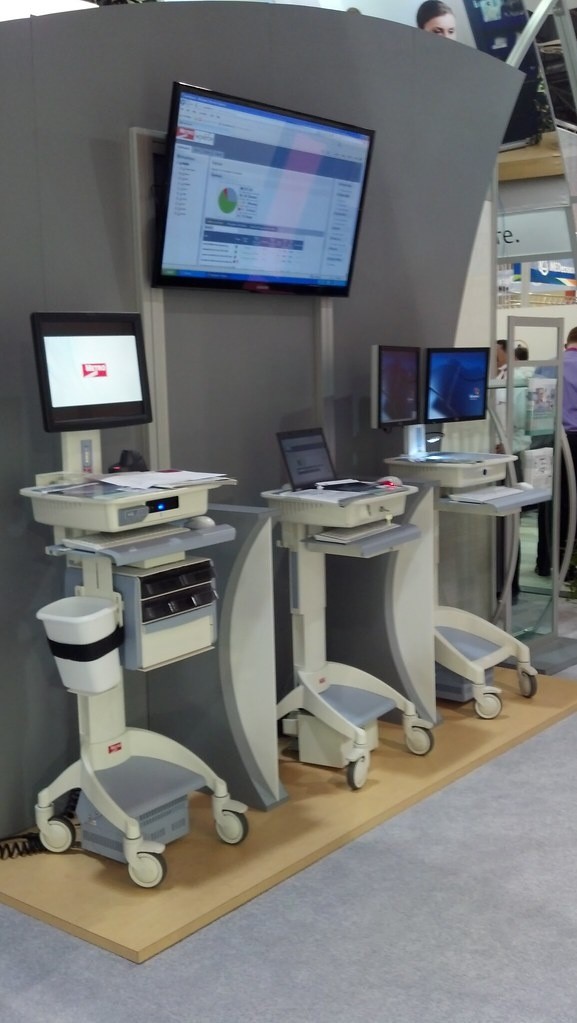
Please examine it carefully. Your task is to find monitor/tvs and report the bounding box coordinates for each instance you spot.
[425,348,491,423]
[150,81,376,298]
[30,311,153,432]
[371,346,420,428]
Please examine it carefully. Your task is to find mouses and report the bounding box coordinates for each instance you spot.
[514,482,534,491]
[184,515,216,531]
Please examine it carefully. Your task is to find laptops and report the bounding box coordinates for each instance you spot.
[276,428,338,493]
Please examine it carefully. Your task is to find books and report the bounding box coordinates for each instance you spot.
[449,485,523,504]
[313,521,400,544]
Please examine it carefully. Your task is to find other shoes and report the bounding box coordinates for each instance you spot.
[534,565,550,576]
[565,568,577,581]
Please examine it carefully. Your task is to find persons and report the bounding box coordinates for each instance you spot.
[496,326,577,605]
[417,0,457,42]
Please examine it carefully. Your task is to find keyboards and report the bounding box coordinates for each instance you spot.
[448,485,524,503]
[315,521,401,544]
[63,523,191,553]
